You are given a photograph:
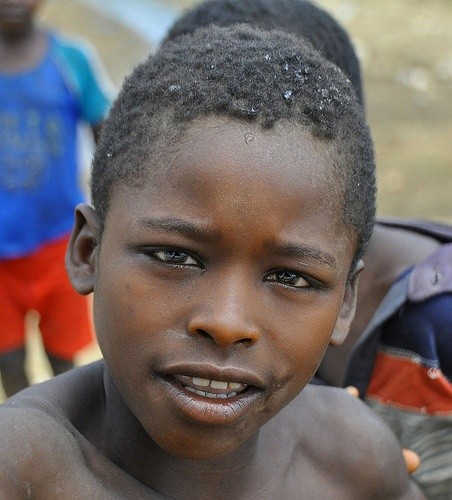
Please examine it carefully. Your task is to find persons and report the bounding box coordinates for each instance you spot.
[0,23,428,500]
[0,0,115,399]
[157,1,452,500]
